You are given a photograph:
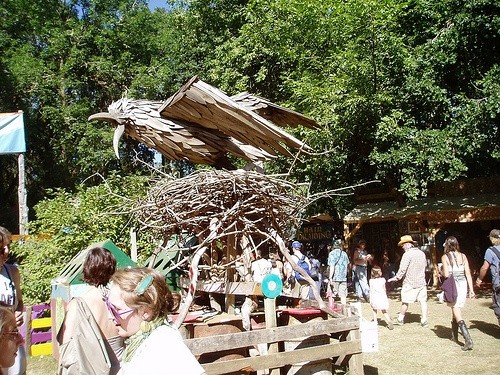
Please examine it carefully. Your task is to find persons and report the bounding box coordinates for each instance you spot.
[254,241,323,301]
[352,240,371,302]
[475,229,500,328]
[369,265,393,329]
[53,247,126,375]
[437,236,475,351]
[389,235,428,328]
[107,267,208,375]
[0,227,28,375]
[327,240,351,305]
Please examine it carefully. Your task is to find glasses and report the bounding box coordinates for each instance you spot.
[105,296,137,324]
[2,330,19,342]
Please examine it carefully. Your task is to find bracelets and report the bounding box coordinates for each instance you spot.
[476,278,482,283]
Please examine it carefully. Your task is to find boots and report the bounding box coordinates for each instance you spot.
[450,322,458,341]
[457,320,473,351]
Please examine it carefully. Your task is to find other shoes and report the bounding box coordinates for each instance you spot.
[392,319,404,326]
[365,296,370,303]
[357,298,366,303]
[421,319,429,327]
[385,319,394,330]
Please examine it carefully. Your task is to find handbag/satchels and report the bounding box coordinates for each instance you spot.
[440,274,458,303]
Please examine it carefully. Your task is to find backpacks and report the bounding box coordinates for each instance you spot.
[293,254,311,281]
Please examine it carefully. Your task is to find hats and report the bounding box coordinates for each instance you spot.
[292,241,301,248]
[486,229,499,238]
[398,235,419,247]
[359,239,366,243]
